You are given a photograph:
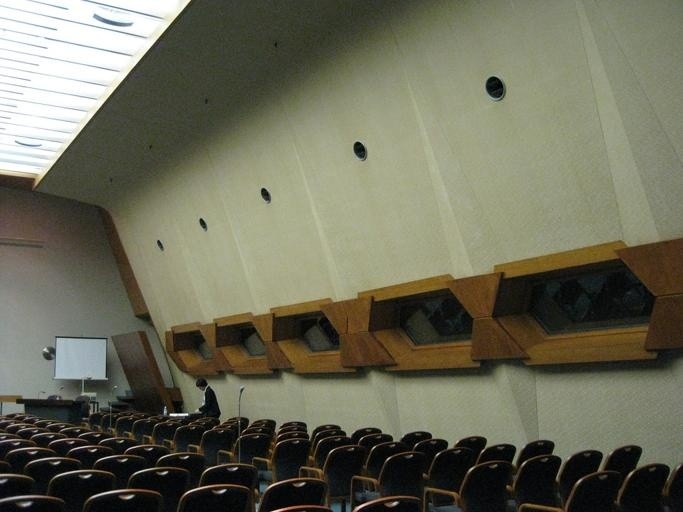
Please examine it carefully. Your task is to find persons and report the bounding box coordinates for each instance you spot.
[196,378,221,418]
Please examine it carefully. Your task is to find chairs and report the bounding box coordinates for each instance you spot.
[0,403,683,512]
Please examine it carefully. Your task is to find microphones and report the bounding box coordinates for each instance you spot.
[37,390,46,399]
[112,383,119,390]
[56,385,65,392]
[240,384,245,393]
